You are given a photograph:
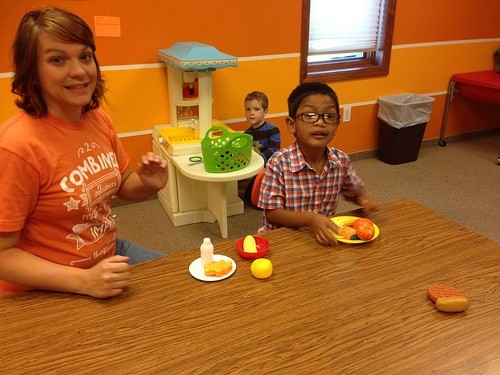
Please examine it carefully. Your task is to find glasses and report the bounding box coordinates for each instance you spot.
[291,111,341,124]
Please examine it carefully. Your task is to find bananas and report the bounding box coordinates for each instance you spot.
[243,234,257,253]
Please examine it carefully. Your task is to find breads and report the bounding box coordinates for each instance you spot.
[204,260,232,276]
[353,218,375,240]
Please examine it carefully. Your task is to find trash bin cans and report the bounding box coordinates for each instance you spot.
[377,93,435,166]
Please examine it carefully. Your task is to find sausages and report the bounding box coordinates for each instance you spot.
[435,296,469,312]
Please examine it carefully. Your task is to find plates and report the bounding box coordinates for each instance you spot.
[327,215,380,244]
[188,254,236,282]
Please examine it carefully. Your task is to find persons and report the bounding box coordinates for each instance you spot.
[243,91,280,209]
[256,82,383,248]
[0,6,169,298]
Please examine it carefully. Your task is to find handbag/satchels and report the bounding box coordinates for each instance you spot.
[201,126,253,173]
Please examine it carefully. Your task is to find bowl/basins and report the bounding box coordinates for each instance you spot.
[234,236,270,259]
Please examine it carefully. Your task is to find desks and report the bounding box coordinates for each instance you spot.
[438,70,500,163]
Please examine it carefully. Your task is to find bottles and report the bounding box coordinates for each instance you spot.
[200,237,214,264]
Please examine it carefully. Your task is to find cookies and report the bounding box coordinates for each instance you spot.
[428,284,464,302]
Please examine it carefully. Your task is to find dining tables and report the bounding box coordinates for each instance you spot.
[0,198,500,375]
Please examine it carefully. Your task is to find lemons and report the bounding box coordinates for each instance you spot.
[251,258,273,279]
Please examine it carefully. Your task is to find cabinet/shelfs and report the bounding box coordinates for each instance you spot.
[151,42,264,238]
